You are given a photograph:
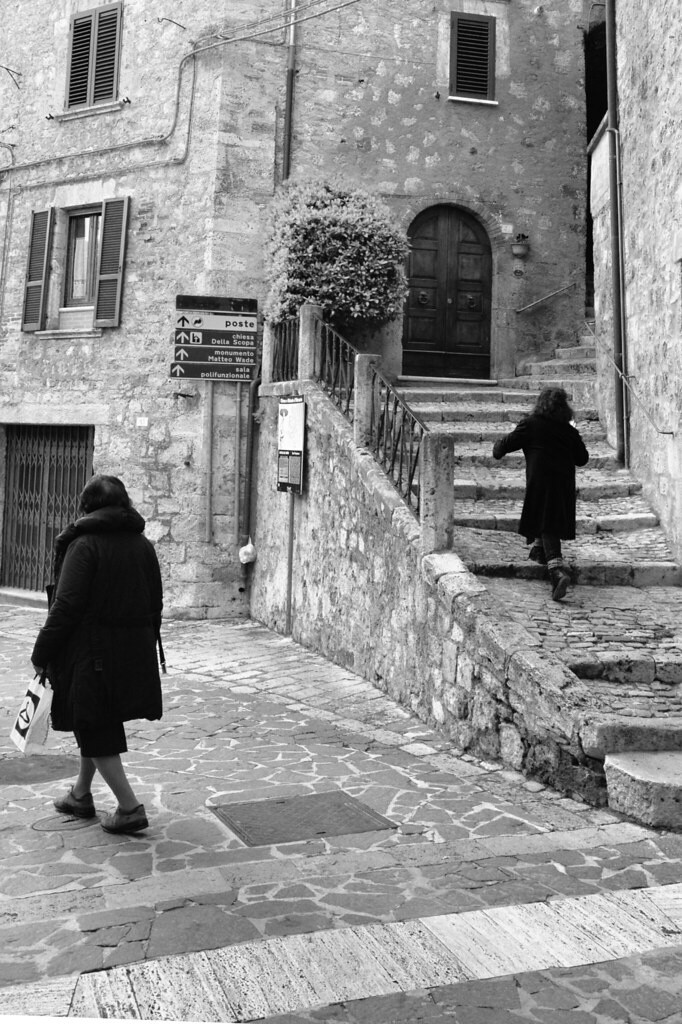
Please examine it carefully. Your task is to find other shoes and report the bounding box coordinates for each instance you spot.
[550,568,571,601]
[54,791,95,818]
[101,804,149,835]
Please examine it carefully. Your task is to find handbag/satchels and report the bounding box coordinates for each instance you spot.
[9,667,54,755]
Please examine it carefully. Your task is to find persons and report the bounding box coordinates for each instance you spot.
[493,386,590,602]
[31,473,163,833]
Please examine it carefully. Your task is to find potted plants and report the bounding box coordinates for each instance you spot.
[511,233,531,258]
